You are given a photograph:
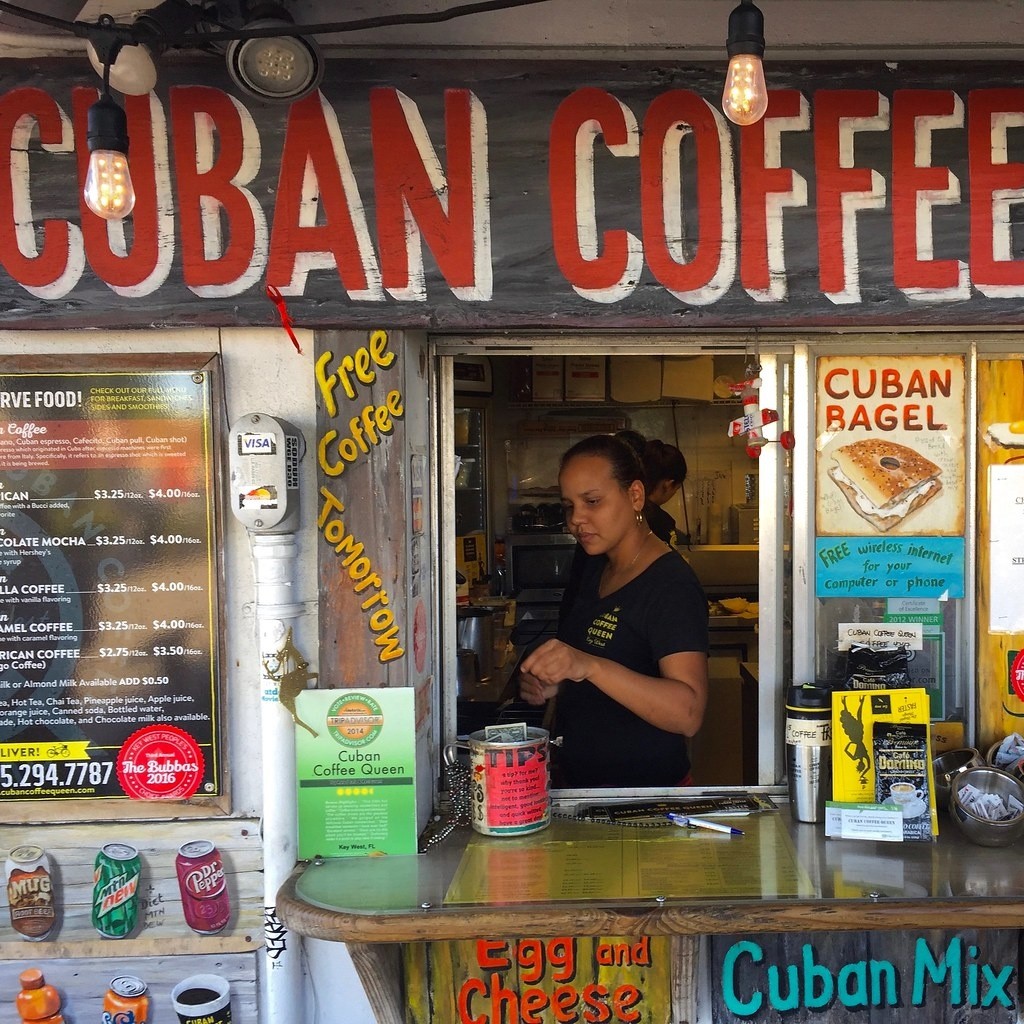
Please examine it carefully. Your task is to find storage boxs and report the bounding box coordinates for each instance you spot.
[468,722,557,838]
[948,766,1024,847]
[931,748,986,810]
[986,734,1024,782]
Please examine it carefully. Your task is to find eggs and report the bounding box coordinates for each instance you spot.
[988,420,1024,446]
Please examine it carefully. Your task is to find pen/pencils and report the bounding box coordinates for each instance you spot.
[665,813,746,836]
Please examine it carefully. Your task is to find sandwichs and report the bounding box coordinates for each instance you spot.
[826,438,943,532]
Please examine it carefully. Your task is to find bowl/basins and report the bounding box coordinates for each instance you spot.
[932,748,988,812]
[947,768,1024,847]
[985,737,1017,770]
[1005,755,1023,785]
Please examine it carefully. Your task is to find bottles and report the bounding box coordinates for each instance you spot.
[15,969,65,1024]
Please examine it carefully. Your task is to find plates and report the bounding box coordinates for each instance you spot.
[881,796,927,819]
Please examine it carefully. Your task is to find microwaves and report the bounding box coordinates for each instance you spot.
[502,532,578,604]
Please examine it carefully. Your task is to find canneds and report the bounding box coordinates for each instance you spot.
[4,839,232,1024]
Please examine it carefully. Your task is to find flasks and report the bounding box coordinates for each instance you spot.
[784,681,833,825]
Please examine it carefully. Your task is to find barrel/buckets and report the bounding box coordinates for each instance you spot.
[459,608,494,681]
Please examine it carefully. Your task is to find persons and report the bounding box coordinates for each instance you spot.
[517,432,710,788]
[568,440,689,576]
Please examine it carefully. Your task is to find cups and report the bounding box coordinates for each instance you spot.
[444,726,553,837]
[889,782,924,807]
[455,648,476,702]
